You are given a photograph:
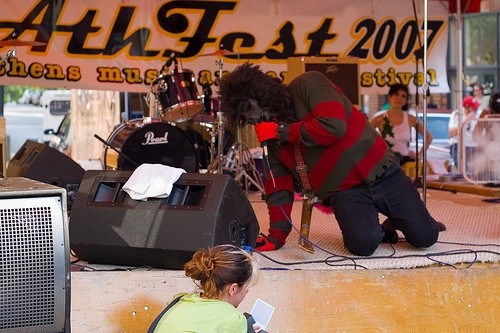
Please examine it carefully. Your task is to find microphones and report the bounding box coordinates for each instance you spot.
[159,52,175,74]
[258,117,269,157]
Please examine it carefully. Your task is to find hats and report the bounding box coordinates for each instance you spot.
[463,95,480,109]
[489,92,500,114]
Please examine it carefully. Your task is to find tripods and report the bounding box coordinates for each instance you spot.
[205,60,267,200]
[412,0,458,193]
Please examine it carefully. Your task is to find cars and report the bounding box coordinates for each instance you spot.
[407,112,452,149]
[43,109,71,155]
[22,88,70,133]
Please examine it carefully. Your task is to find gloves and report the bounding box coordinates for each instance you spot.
[255,121,283,143]
[255,236,276,252]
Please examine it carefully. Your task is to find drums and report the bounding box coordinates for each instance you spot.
[194,91,229,125]
[99,116,201,172]
[153,69,202,121]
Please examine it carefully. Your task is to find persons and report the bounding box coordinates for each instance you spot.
[147,244,261,333]
[448,95,487,175]
[217,60,446,257]
[369,81,434,184]
[472,91,500,176]
[470,82,490,117]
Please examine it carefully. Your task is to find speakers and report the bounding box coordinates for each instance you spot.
[7,140,85,211]
[287,57,361,111]
[70,170,261,269]
[0,177,72,333]
